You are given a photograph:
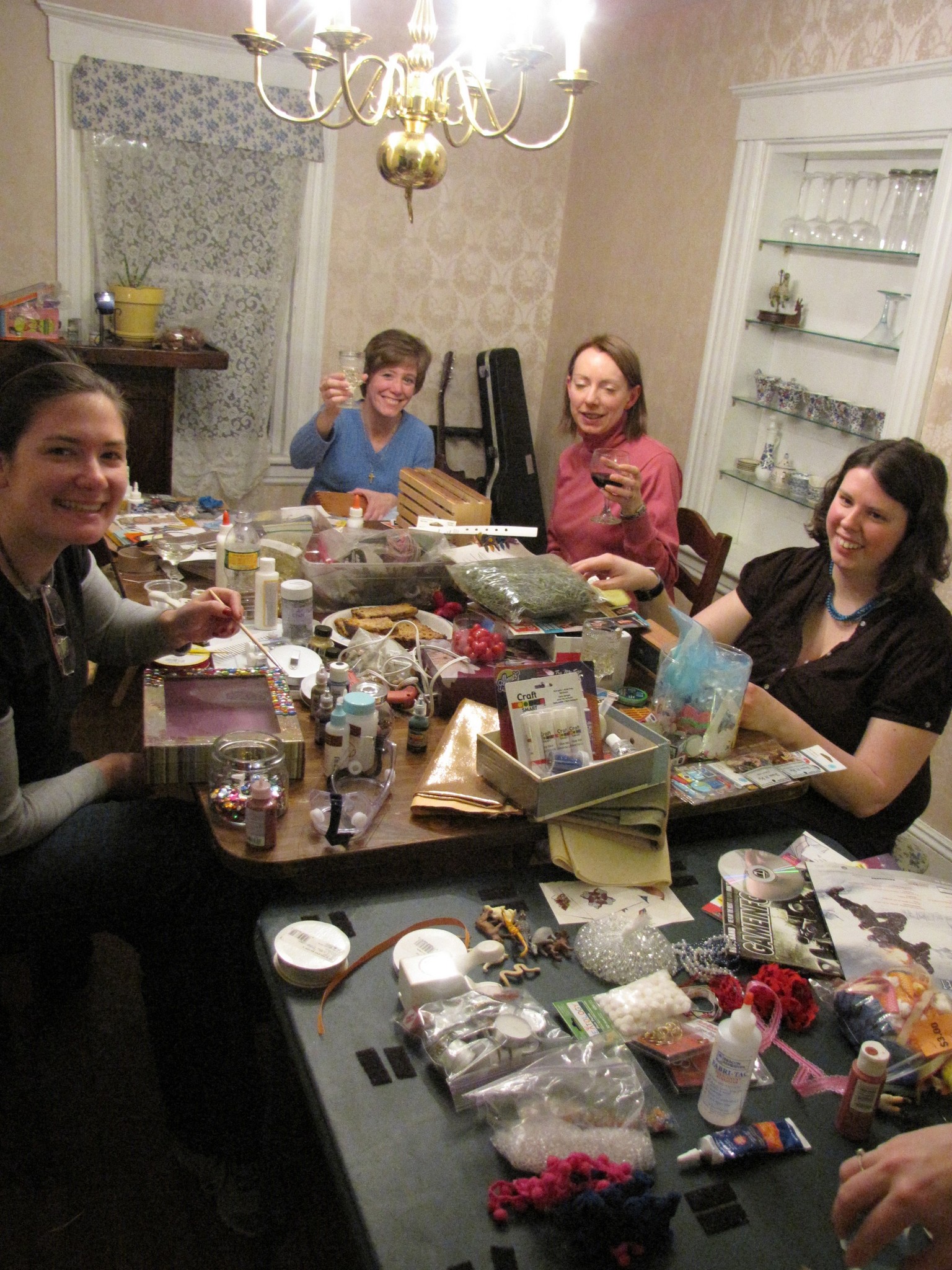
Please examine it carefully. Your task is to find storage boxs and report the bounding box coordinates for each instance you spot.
[143,667,304,788]
[0,282,59,342]
[476,706,669,822]
[415,636,551,714]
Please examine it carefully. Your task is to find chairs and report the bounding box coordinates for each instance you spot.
[676,508,733,618]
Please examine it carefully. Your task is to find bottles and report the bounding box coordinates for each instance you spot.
[407,695,429,753]
[118,467,144,514]
[871,168,939,254]
[698,992,762,1127]
[755,422,779,481]
[215,510,313,666]
[208,730,290,852]
[834,1040,890,1141]
[346,495,364,528]
[310,663,394,780]
[606,734,638,759]
[307,625,345,669]
[582,698,593,746]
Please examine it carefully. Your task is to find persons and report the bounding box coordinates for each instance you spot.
[543,336,682,615]
[0,338,315,1241]
[558,435,951,860]
[289,328,435,521]
[830,1122,952,1270]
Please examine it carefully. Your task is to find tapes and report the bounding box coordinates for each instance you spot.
[117,544,160,574]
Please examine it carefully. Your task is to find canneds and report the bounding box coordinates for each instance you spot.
[280,579,313,645]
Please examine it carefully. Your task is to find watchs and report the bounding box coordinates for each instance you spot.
[620,503,645,521]
[633,567,663,601]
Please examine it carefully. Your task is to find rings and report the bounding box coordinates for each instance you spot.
[857,1148,865,1171]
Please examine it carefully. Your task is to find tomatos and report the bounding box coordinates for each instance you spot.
[432,591,462,621]
[452,623,506,663]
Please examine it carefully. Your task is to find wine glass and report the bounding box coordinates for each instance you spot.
[579,618,622,687]
[152,531,199,580]
[336,350,365,409]
[861,290,911,349]
[589,448,630,525]
[778,170,884,249]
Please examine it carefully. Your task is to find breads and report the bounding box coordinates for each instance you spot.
[334,603,449,650]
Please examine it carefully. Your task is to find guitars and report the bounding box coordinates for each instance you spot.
[435,351,466,480]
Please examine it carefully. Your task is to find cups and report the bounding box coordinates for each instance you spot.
[144,579,188,610]
[547,750,590,774]
[770,453,826,504]
[755,374,886,439]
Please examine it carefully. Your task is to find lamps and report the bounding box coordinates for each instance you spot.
[230,0,601,224]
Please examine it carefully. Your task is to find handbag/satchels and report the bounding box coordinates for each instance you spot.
[645,606,753,759]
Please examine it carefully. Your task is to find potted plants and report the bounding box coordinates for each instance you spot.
[106,247,165,345]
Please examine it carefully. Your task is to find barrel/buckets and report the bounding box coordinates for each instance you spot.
[651,640,753,761]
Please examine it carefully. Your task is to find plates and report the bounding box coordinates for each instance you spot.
[737,458,760,475]
[322,606,453,647]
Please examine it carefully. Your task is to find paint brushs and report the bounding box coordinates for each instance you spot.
[207,588,287,679]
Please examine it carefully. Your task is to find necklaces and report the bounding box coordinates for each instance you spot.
[825,560,888,623]
[359,403,402,484]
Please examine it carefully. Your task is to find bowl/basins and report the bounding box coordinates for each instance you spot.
[451,613,509,663]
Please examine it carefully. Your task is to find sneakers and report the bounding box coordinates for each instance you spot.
[172,1136,279,1237]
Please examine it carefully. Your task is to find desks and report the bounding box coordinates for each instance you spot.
[116,572,952,1270]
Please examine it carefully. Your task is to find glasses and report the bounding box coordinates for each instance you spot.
[39,584,77,675]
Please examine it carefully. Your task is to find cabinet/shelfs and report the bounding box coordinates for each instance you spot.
[79,342,229,494]
[674,57,952,594]
